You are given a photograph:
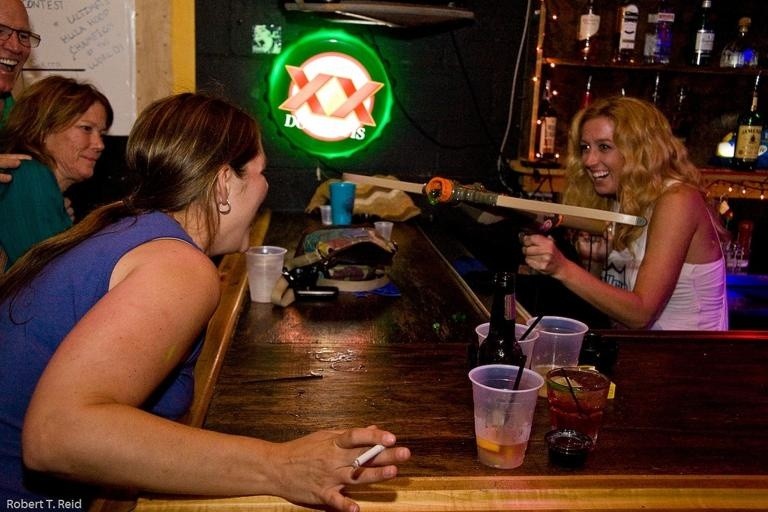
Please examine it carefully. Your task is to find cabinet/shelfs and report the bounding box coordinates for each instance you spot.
[508,1,768,204]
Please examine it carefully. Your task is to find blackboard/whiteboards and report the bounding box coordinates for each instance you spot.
[11,0,137,136]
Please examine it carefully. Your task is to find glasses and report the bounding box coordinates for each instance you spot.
[0,23,41,48]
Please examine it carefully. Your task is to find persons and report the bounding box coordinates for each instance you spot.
[0,94,411,512]
[0,75,114,280]
[0,1,78,222]
[518,95,730,333]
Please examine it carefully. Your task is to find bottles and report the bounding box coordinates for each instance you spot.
[477,270,523,365]
[535,0,768,173]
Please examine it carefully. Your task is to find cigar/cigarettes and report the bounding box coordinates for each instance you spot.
[352,442,386,469]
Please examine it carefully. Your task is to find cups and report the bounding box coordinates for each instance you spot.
[321,182,356,226]
[245,245,287,303]
[374,221,394,240]
[470,315,611,471]
